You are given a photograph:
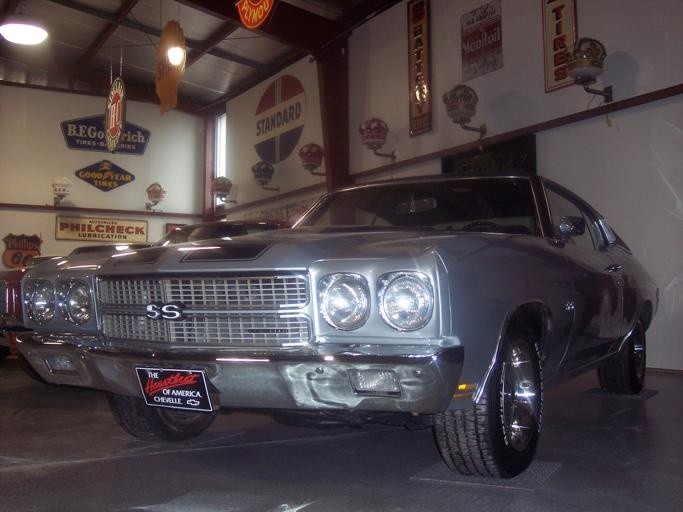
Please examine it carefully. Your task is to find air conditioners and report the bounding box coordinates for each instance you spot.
[0,0,49,46]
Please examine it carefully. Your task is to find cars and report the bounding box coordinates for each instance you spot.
[68,243,154,255]
[11,172,660,479]
[0,217,284,381]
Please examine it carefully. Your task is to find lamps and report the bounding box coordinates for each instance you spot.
[248,159,282,193]
[141,182,167,207]
[562,39,612,108]
[297,140,332,180]
[210,175,236,205]
[354,116,400,165]
[167,0,187,67]
[53,176,74,207]
[437,81,494,146]
